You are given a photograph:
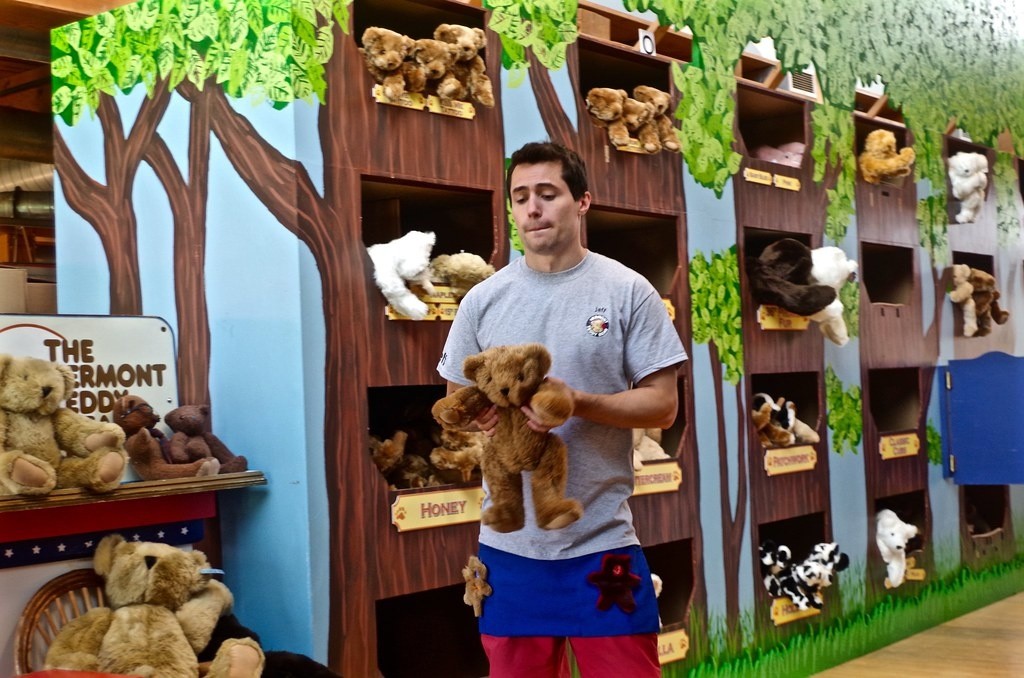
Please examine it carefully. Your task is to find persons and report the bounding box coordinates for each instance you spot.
[439,141,688,678]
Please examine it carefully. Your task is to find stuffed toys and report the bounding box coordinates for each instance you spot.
[632,429,670,468]
[949,264,1009,336]
[949,150,988,222]
[858,128,916,183]
[43,534,264,678]
[650,574,663,628]
[164,405,248,473]
[743,238,858,346]
[369,425,488,491]
[757,141,806,168]
[366,230,495,320]
[588,85,681,152]
[762,542,849,610]
[114,395,220,479]
[430,345,580,528]
[876,509,918,587]
[358,23,496,108]
[752,393,821,448]
[0,354,128,495]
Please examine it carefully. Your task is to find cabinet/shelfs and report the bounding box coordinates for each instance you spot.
[0,0,1024,678]
